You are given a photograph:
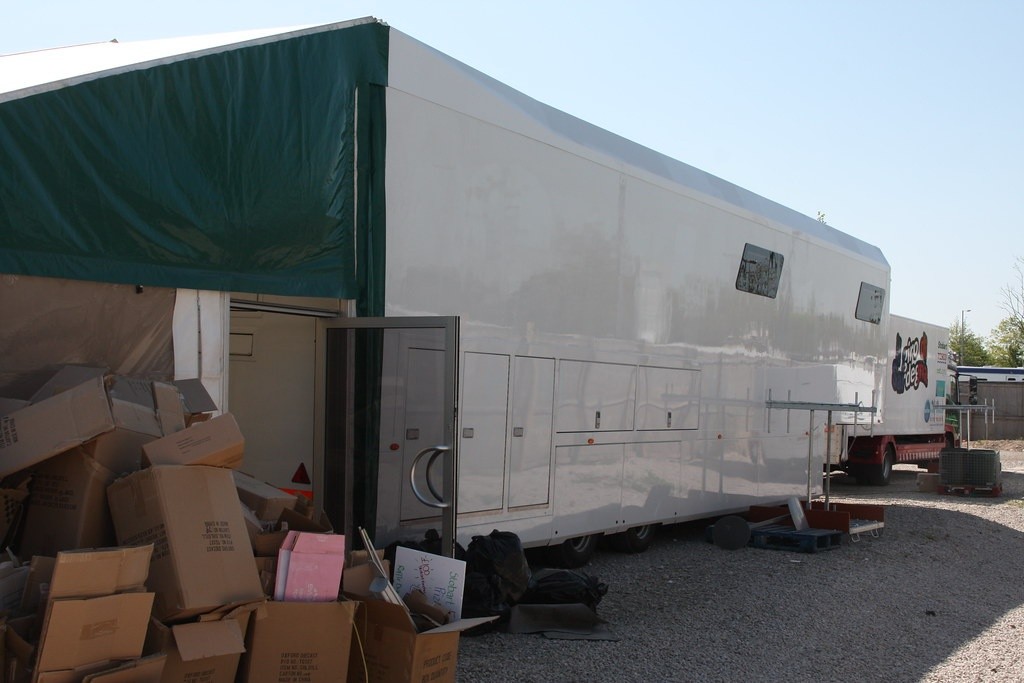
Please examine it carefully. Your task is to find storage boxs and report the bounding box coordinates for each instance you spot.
[0,364,501,683]
[940,448,1001,485]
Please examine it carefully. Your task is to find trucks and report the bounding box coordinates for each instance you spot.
[845,313,961,485]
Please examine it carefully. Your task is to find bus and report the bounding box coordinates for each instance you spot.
[957,365,1024,383]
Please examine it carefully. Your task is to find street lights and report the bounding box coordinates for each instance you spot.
[961,310,971,366]
